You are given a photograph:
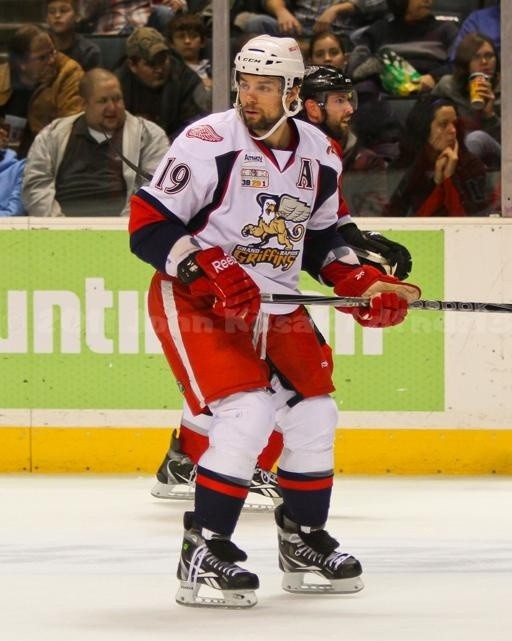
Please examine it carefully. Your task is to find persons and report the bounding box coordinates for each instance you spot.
[156,64,412,498]
[129,33,421,591]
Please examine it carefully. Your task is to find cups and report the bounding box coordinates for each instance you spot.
[467,73,489,109]
[2,115,28,147]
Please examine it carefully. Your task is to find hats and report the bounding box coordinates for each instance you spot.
[124,26,171,64]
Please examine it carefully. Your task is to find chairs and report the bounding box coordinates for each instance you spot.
[0,0,500,216]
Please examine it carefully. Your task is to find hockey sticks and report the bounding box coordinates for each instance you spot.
[97,123,392,266]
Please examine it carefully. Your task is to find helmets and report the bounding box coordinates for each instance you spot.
[231,31,308,89]
[298,60,356,105]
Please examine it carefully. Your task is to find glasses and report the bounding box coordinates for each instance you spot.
[22,47,59,67]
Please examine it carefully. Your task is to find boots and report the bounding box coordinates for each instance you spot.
[173,508,264,593]
[246,461,289,500]
[151,428,198,491]
[272,503,365,581]
